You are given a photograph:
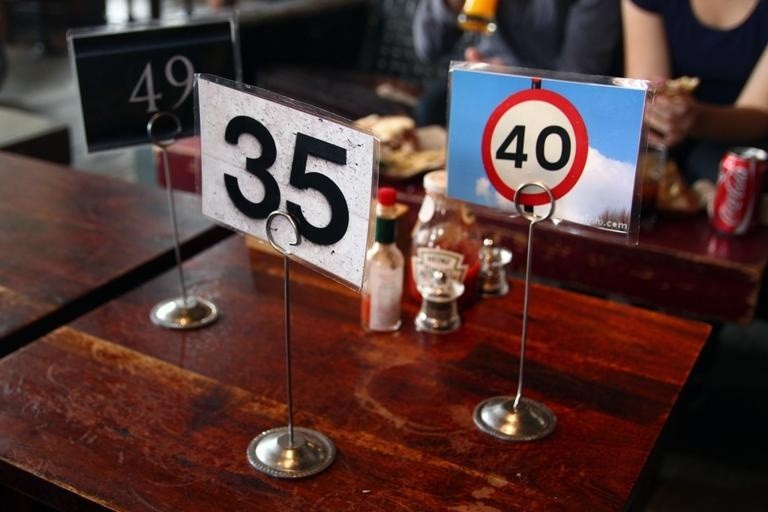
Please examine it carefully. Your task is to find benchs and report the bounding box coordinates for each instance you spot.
[228,0,430,127]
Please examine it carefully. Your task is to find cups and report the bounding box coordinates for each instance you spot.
[451,2,495,48]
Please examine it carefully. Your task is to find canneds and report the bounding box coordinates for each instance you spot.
[709,146,767,235]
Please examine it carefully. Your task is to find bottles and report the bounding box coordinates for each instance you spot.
[362,185,404,333]
[409,169,482,311]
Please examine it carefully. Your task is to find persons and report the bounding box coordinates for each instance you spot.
[621,1,768,152]
[201,1,234,16]
[379,1,622,172]
[380,0,441,106]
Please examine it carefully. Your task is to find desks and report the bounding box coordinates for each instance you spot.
[0,99,79,172]
[0,234,714,511]
[0,144,228,359]
